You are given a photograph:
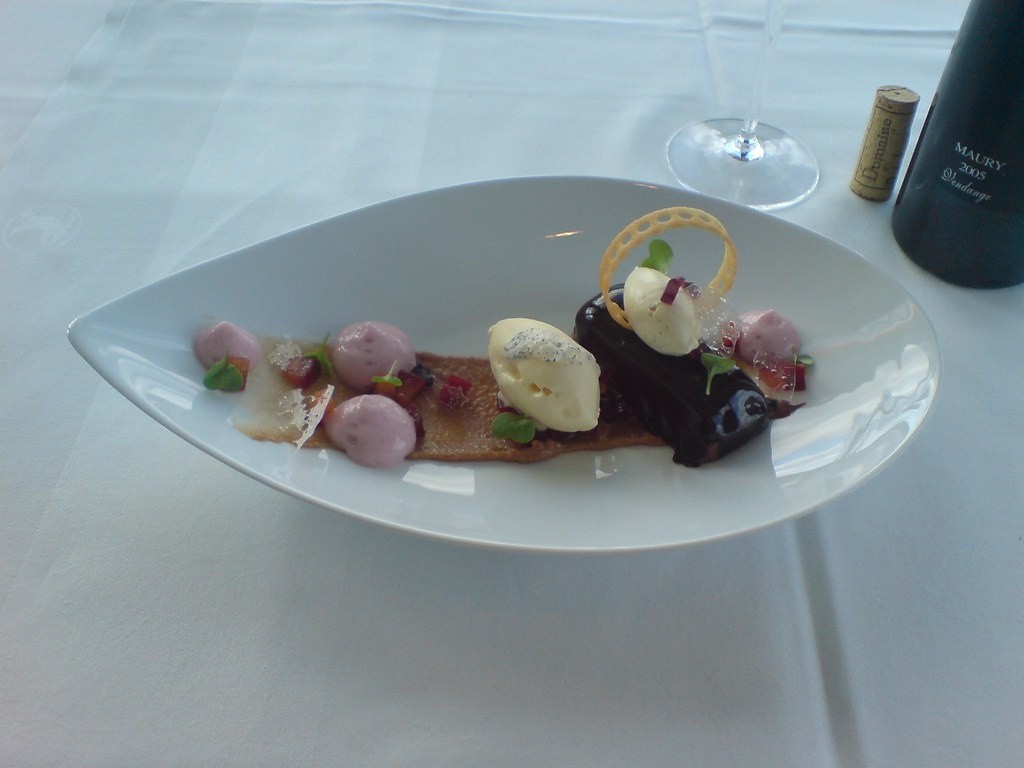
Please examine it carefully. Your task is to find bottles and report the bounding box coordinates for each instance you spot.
[891,0,1024,290]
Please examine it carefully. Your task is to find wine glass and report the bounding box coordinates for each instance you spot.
[665,0,820,210]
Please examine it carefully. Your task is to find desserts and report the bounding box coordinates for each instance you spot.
[196,207,813,470]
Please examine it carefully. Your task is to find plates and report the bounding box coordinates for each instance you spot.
[68,175,941,553]
